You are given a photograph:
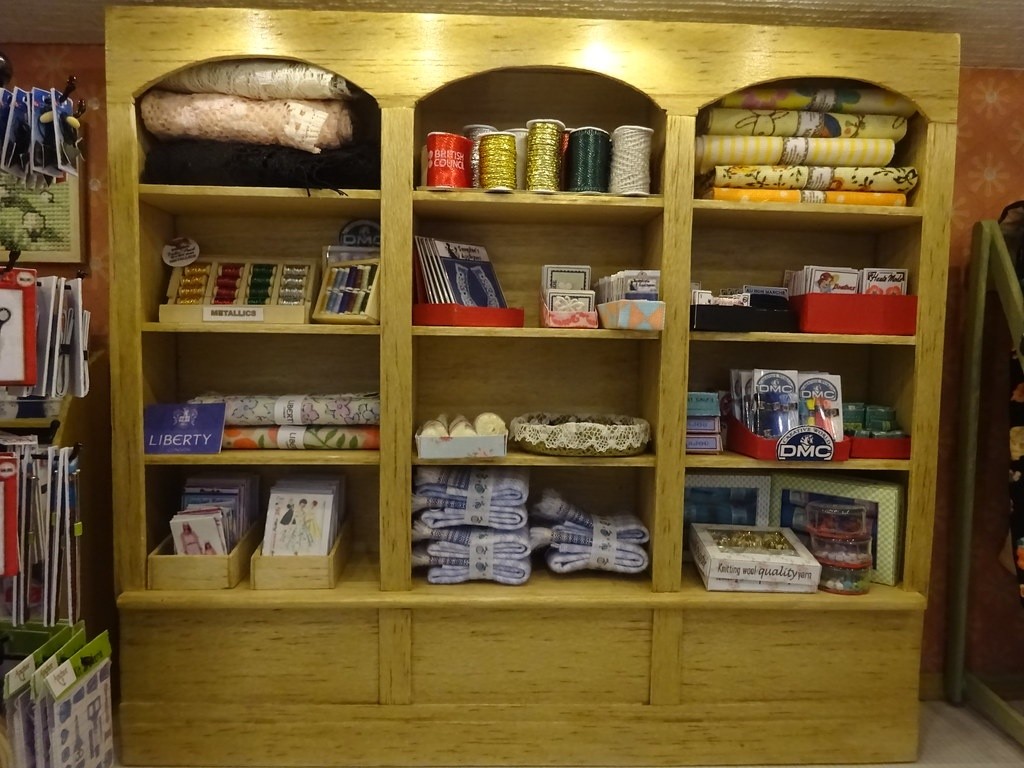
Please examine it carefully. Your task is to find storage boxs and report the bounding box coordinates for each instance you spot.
[686,392,724,454]
[414,253,526,328]
[682,472,772,562]
[789,293,918,336]
[249,515,351,591]
[538,290,598,329]
[596,300,667,331]
[311,258,380,325]
[688,523,823,593]
[691,304,793,332]
[771,469,905,594]
[416,429,508,458]
[727,410,911,460]
[159,255,322,325]
[147,518,259,591]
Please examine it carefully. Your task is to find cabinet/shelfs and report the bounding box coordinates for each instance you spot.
[106,0,963,768]
